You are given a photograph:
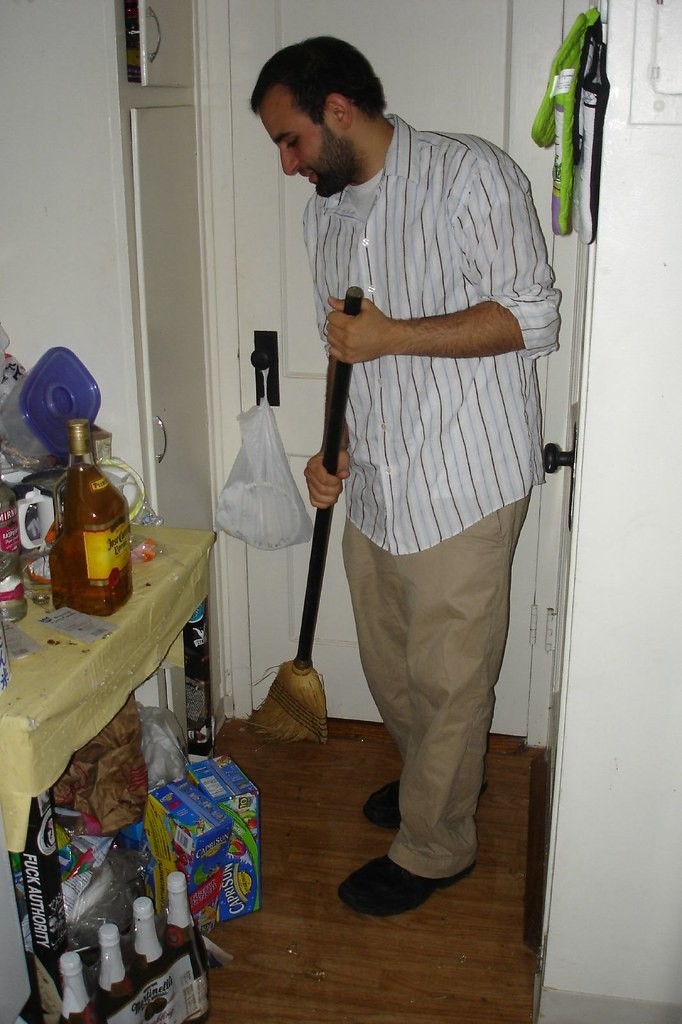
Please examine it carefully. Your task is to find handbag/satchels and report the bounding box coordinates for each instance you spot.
[213,367,313,552]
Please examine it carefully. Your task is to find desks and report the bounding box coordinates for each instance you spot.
[0,524,216,1024]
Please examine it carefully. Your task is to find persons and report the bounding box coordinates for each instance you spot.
[249,38,562,917]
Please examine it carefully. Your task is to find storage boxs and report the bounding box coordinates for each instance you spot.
[143,778,234,935]
[0,346,101,457]
[185,752,262,924]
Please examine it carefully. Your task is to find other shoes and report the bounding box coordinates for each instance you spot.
[363,777,488,829]
[337,854,477,917]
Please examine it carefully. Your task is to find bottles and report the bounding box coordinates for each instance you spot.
[0,478,28,624]
[49,416,134,618]
[57,872,212,1024]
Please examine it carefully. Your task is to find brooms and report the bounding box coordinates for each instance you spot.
[244,284,364,754]
